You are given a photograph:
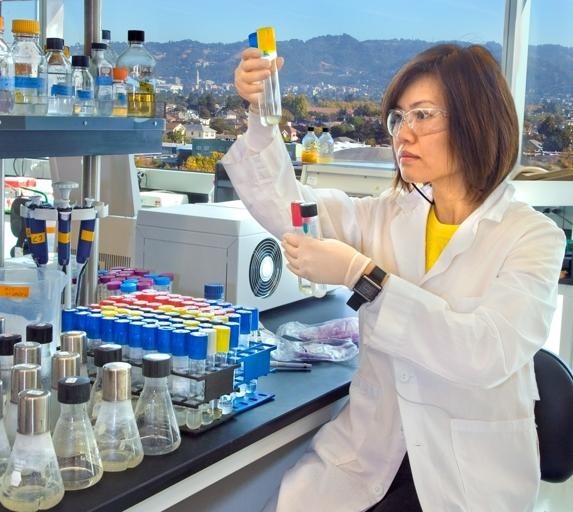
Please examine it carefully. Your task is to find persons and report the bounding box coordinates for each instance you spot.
[220,42,567,511]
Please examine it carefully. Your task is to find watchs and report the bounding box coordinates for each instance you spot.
[345,265,387,311]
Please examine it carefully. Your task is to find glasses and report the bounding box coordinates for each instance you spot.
[386,107,449,137]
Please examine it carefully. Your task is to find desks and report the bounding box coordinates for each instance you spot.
[0,286,362,512]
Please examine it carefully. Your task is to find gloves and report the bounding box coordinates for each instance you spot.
[234,48,285,113]
[281,231,372,292]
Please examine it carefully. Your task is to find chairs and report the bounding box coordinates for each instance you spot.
[368,350,573,511]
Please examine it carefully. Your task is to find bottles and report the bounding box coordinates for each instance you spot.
[61,267,243,331]
[4,363,42,452]
[111,67,128,117]
[0,15,15,115]
[102,30,119,68]
[246,306,259,393]
[221,322,240,415]
[186,331,208,430]
[10,20,48,116]
[90,42,113,117]
[292,201,315,296]
[256,27,282,125]
[44,45,70,58]
[85,315,102,382]
[114,319,131,363]
[0,388,65,512]
[116,30,157,117]
[213,325,230,420]
[200,329,216,426]
[51,376,104,490]
[60,330,92,393]
[128,321,146,392]
[93,362,144,471]
[236,309,253,398]
[300,202,326,298]
[50,350,80,437]
[0,379,12,476]
[0,332,21,416]
[316,127,335,164]
[87,344,124,430]
[157,327,175,393]
[45,38,73,117]
[70,55,95,118]
[134,352,181,455]
[301,127,319,165]
[26,321,53,391]
[6,341,42,406]
[249,32,272,128]
[142,324,158,355]
[171,329,191,426]
